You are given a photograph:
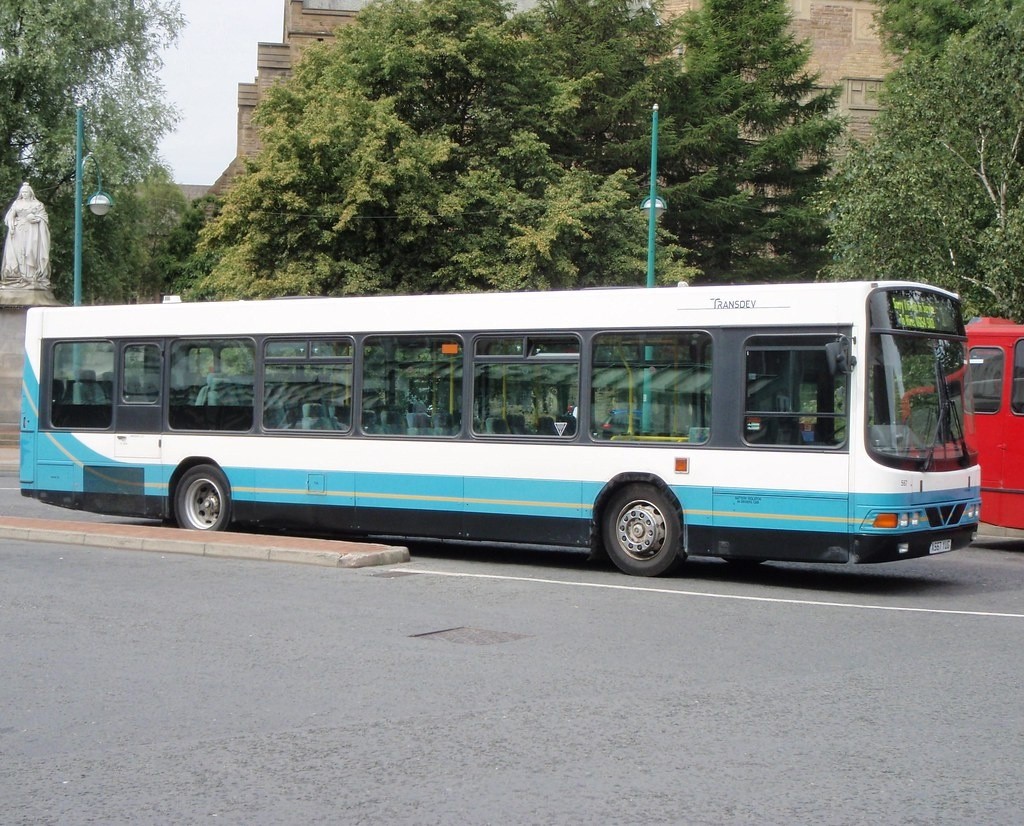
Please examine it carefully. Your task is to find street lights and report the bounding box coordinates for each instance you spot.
[637,102,669,436]
[73,99,116,404]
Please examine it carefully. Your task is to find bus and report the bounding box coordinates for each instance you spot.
[897,311,1022,547]
[17,278,985,578]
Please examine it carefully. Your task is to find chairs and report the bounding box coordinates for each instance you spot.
[71,369,578,436]
